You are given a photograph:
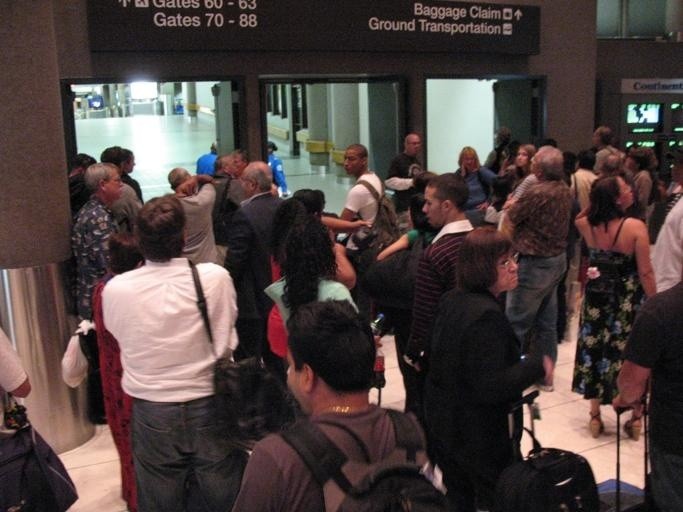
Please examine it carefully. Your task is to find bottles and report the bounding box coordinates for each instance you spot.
[373,344,385,389]
[371,313,384,335]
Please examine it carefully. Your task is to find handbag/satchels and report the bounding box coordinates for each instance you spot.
[361,253,422,311]
[77,318,100,370]
[211,356,270,449]
[0,425,80,512]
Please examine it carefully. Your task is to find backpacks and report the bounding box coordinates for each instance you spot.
[585,258,620,308]
[279,408,453,512]
[372,196,399,244]
[213,198,241,245]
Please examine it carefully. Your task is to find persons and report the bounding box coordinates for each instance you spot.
[0,323,33,512]
[68,128,682,512]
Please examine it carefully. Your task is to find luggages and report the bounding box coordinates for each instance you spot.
[591,410,652,511]
[493,446,603,512]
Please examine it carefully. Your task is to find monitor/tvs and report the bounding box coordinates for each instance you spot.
[668,140,683,148]
[627,102,662,134]
[625,141,660,156]
[671,102,683,133]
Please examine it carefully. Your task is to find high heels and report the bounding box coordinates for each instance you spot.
[589,413,605,438]
[622,415,643,442]
